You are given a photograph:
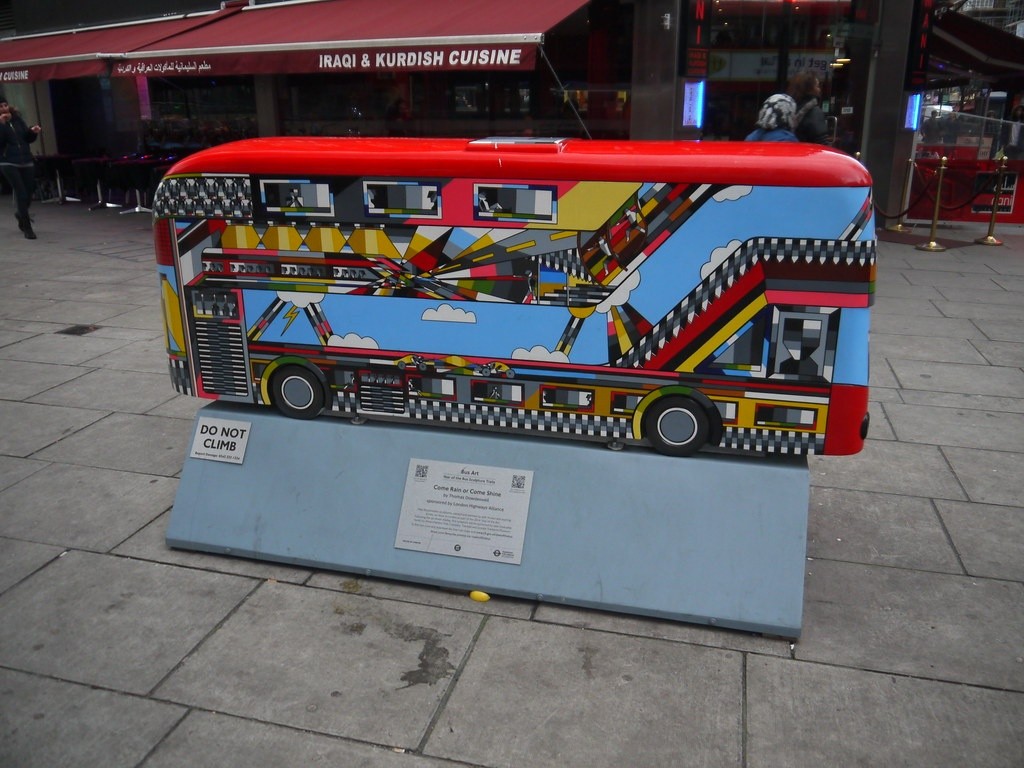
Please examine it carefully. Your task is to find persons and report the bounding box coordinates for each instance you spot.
[920,104,1024,159]
[745,92,799,141]
[787,69,833,146]
[0,93,43,240]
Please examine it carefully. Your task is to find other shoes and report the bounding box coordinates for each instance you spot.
[15,210,37,240]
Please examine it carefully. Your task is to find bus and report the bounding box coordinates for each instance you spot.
[152,137,878,456]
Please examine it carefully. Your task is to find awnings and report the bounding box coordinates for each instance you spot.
[925,8,1024,90]
[110,0,594,79]
[0,0,247,82]
